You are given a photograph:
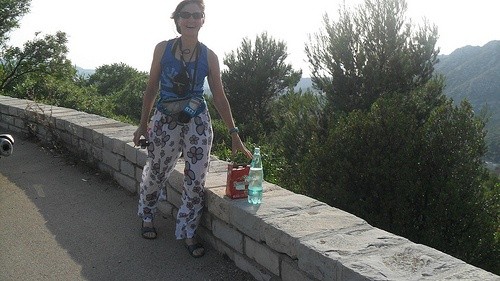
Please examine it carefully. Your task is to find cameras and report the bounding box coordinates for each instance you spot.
[138,139,150,149]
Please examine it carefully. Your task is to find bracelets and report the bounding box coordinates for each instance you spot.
[228,127,239,135]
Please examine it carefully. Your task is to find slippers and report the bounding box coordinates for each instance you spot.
[183,239,205,258]
[141,220,157,239]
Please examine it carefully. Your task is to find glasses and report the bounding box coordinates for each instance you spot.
[178,11,203,19]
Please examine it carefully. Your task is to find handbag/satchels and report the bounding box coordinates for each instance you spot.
[226,162,250,199]
[157,94,203,123]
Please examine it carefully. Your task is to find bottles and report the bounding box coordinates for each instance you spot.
[248,148,263,204]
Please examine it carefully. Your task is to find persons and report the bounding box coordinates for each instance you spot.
[133,0,254,258]
